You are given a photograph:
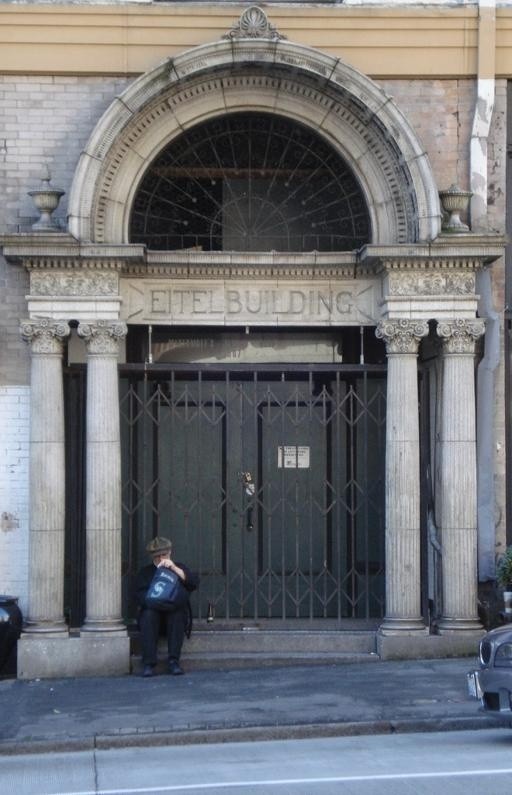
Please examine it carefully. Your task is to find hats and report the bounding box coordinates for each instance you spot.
[146,537,172,558]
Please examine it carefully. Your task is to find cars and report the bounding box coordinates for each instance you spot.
[466,619,512,720]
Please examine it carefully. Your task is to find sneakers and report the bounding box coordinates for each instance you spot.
[167,662,183,674]
[143,668,154,676]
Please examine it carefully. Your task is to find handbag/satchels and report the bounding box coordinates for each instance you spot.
[145,566,188,612]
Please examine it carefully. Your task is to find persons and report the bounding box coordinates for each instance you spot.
[132,536,201,680]
